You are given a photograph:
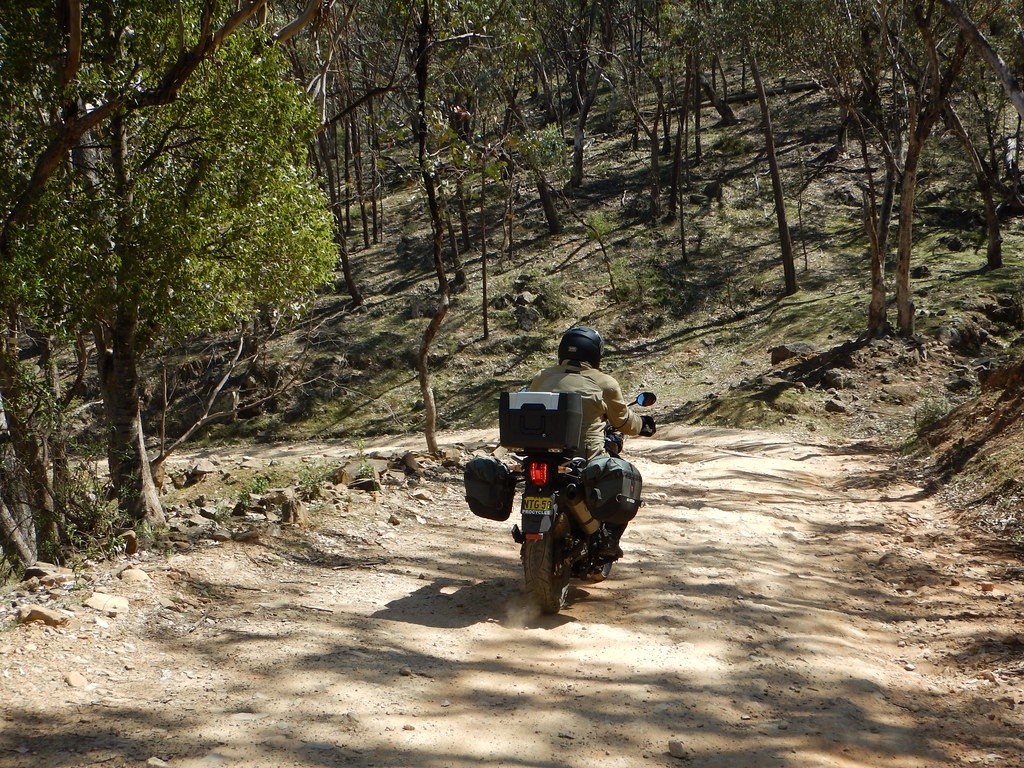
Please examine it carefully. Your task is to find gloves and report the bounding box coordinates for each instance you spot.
[638,416,657,438]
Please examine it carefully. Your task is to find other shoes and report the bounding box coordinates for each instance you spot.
[600,538,623,559]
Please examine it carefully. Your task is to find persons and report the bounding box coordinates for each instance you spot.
[519,327,643,563]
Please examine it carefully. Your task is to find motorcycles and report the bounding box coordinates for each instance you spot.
[500,390,656,613]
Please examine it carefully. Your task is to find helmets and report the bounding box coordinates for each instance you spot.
[558,326,604,368]
